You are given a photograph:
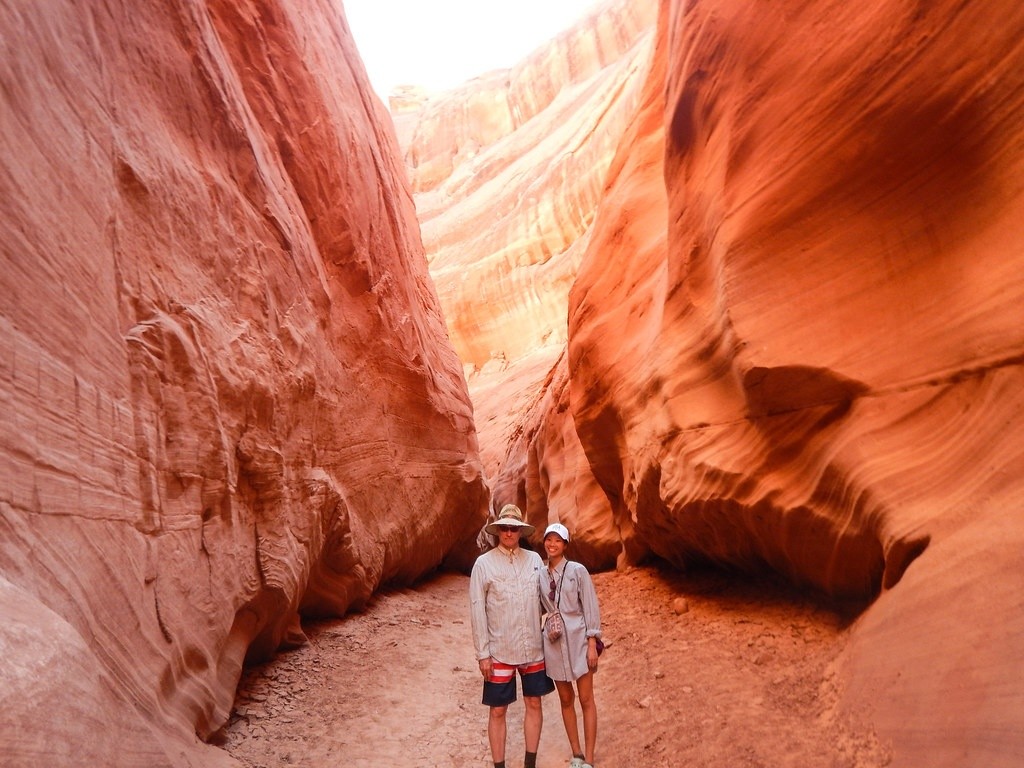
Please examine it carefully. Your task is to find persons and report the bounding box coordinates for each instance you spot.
[540,523,600,768]
[469,504,544,768]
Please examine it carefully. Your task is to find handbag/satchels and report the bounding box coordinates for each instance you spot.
[545,608,564,644]
[596,637,604,656]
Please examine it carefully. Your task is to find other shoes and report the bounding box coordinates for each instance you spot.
[568,756,592,768]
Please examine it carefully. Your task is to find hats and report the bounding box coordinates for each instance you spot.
[485,503,536,537]
[544,523,570,543]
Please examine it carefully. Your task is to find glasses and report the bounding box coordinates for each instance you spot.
[500,525,519,532]
[548,580,556,601]
